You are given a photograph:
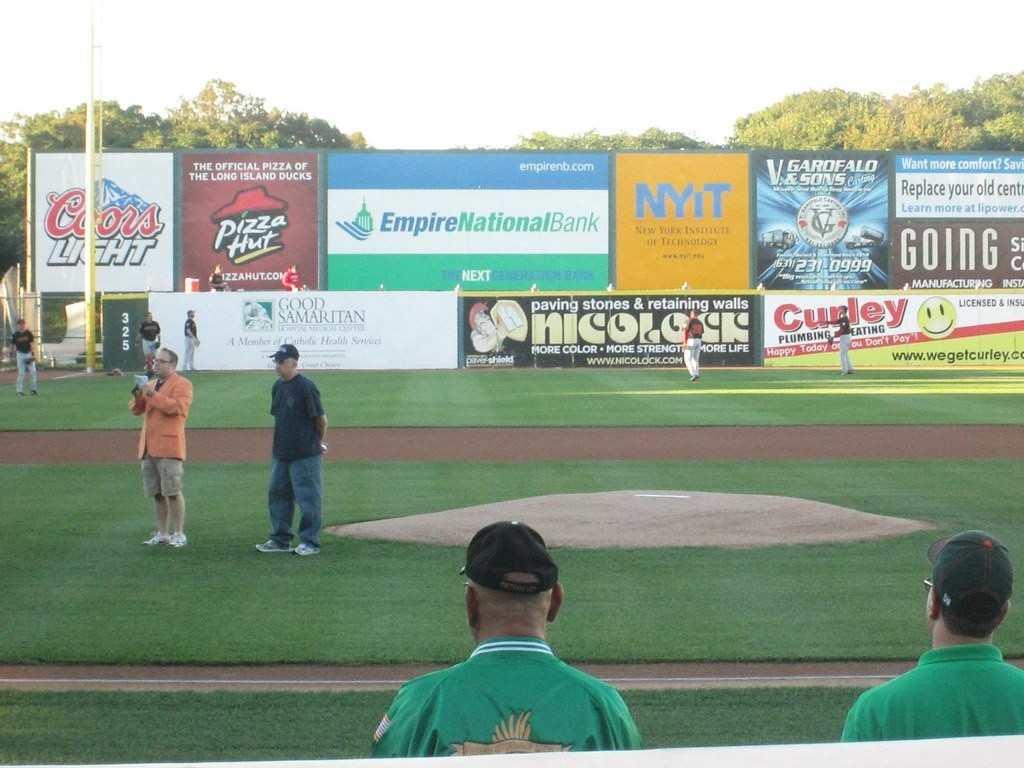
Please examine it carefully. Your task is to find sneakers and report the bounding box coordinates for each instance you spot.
[255,539,292,553]
[292,542,320,556]
[167,534,187,546]
[142,533,171,546]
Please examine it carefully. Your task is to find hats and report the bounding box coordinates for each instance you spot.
[15,318,26,322]
[925,530,1014,619]
[269,344,299,361]
[459,521,559,594]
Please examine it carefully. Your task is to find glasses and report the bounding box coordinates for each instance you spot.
[923,577,948,603]
[273,359,284,364]
[152,358,170,363]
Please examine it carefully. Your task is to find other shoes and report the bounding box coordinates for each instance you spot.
[16,391,26,396]
[148,372,153,378]
[847,370,854,374]
[30,389,39,395]
[840,370,848,375]
[690,375,699,381]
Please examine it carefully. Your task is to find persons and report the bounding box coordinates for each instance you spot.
[130,348,193,548]
[9,319,38,397]
[255,344,327,555]
[183,311,200,372]
[841,529,1024,745]
[209,264,227,293]
[681,310,705,381]
[281,264,303,291]
[372,520,642,759]
[818,306,855,376]
[136,312,161,379]
